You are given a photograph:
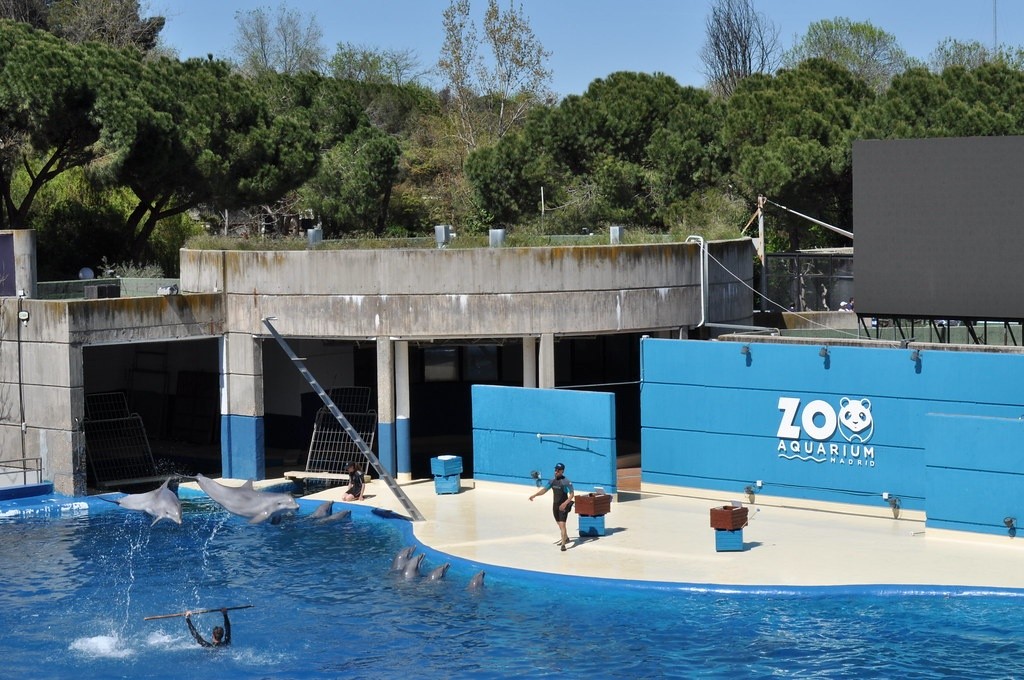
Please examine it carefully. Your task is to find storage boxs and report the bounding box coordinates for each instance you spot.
[434,474,461,495]
[575,493,611,516]
[579,516,605,536]
[717,529,744,551]
[710,506,749,530]
[431,456,463,476]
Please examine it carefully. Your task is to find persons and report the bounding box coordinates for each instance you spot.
[528,462,574,551]
[342,460,365,501]
[838,297,855,312]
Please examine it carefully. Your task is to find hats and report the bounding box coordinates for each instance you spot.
[554,463,565,469]
[343,461,354,469]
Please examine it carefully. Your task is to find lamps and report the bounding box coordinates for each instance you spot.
[530,471,539,478]
[911,350,920,361]
[744,485,761,494]
[889,498,900,510]
[741,342,751,354]
[819,343,829,357]
[1004,517,1017,530]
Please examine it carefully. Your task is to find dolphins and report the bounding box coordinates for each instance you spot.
[391,544,486,591]
[97,472,301,528]
[305,500,352,524]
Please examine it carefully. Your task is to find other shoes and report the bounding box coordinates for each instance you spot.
[561,545,567,551]
